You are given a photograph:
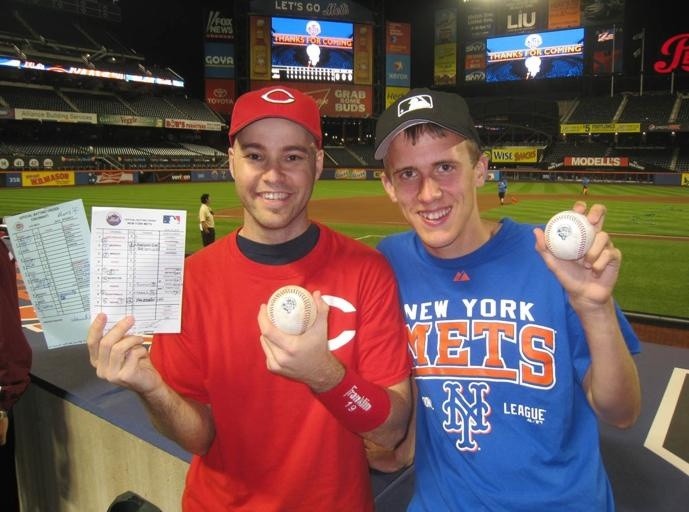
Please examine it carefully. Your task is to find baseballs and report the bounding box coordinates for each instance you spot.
[267,284,317,337]
[543,210,595,260]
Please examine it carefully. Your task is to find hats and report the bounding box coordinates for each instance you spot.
[229,86,323,150]
[373,87,480,161]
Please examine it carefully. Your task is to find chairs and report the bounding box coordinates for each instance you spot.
[0,0,230,170]
[541,94,688,172]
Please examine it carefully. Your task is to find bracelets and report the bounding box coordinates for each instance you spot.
[313,370,390,432]
[0,410,8,421]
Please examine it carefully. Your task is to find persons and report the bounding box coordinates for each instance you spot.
[90,88,414,512]
[200,193,216,245]
[581,177,590,194]
[0,237,32,512]
[499,178,506,204]
[372,91,641,512]
